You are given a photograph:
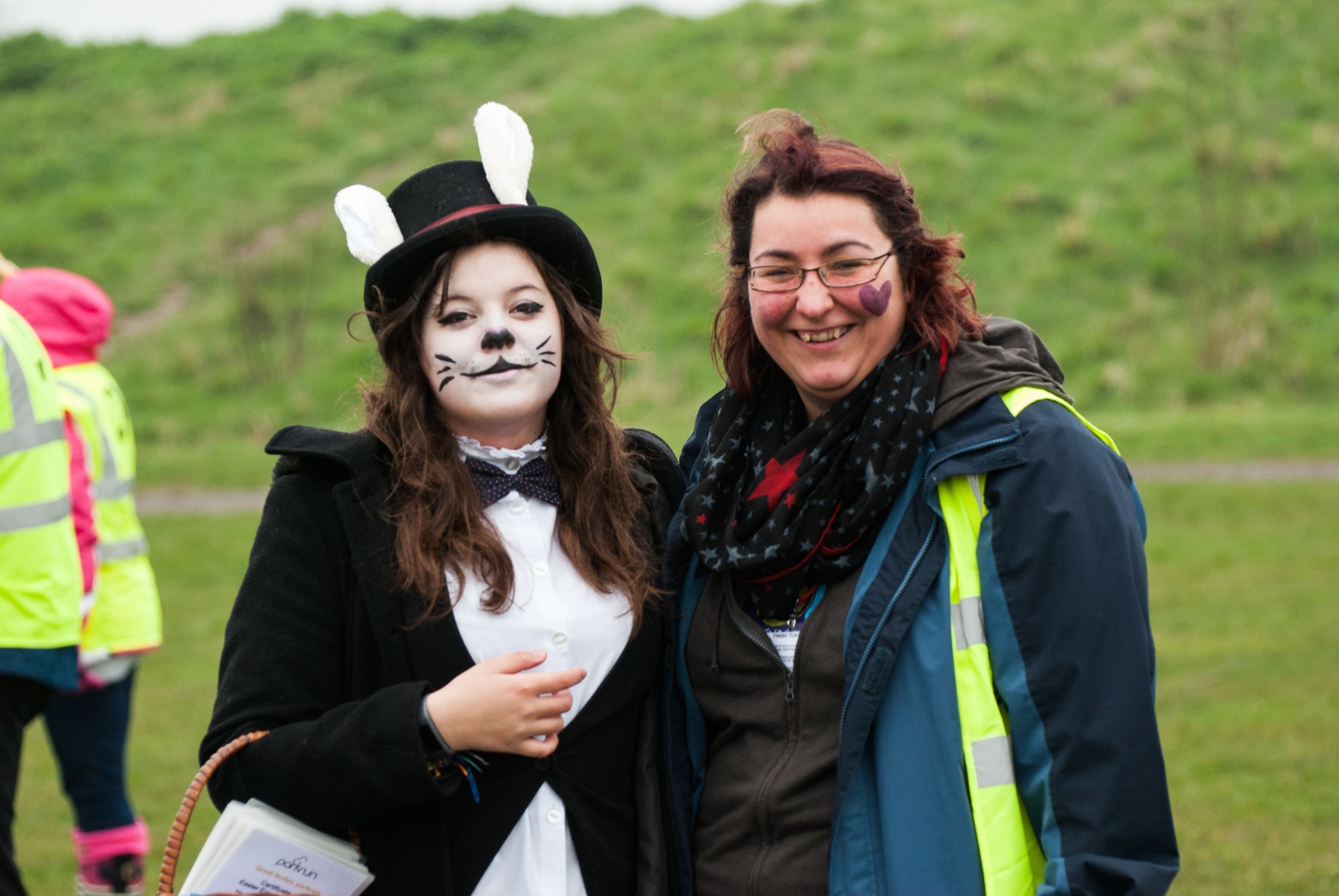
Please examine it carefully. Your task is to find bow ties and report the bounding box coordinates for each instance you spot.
[462,453,563,510]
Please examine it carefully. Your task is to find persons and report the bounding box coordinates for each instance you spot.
[620,105,1184,896]
[0,243,162,896]
[198,103,685,896]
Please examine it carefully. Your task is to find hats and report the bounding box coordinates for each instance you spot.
[335,101,603,364]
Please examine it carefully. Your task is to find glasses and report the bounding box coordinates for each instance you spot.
[747,241,903,293]
